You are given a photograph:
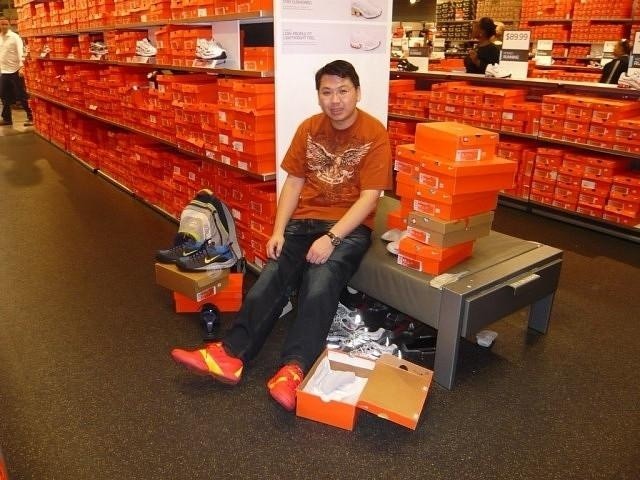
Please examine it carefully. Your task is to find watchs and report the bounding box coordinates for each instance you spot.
[326,232,342,247]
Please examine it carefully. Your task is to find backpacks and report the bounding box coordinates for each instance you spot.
[176,188,243,264]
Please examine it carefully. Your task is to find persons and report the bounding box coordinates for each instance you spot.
[464,17,499,74]
[0,17,33,126]
[599,38,630,84]
[171,60,394,411]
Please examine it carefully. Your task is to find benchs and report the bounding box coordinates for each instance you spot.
[347,195,563,391]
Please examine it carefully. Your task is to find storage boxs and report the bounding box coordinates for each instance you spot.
[293,345,435,432]
[387,0,640,243]
[392,237,475,276]
[14,0,274,271]
[153,259,245,315]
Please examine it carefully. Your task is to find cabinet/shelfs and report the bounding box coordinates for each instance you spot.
[14,0,394,277]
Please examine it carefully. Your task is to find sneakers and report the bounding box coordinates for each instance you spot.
[266,363,305,412]
[617,72,640,90]
[484,63,512,78]
[23,117,33,126]
[155,238,238,272]
[170,340,244,386]
[326,301,402,367]
[195,38,227,59]
[0,119,13,125]
[396,59,418,72]
[89,41,109,54]
[136,38,158,57]
